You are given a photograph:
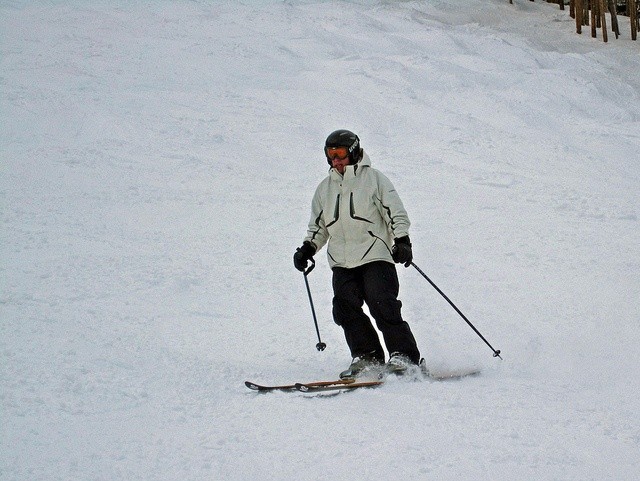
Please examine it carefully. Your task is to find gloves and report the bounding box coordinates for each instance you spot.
[294,240,318,276]
[391,235,413,267]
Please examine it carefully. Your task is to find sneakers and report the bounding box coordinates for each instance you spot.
[387,351,419,374]
[339,354,385,379]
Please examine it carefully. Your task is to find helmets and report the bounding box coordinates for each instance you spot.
[324,130,360,166]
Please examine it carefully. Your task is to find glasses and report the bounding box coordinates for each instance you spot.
[325,139,358,160]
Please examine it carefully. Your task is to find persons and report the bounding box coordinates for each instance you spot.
[294,129,421,383]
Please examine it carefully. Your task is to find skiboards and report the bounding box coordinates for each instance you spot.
[245,367,479,392]
